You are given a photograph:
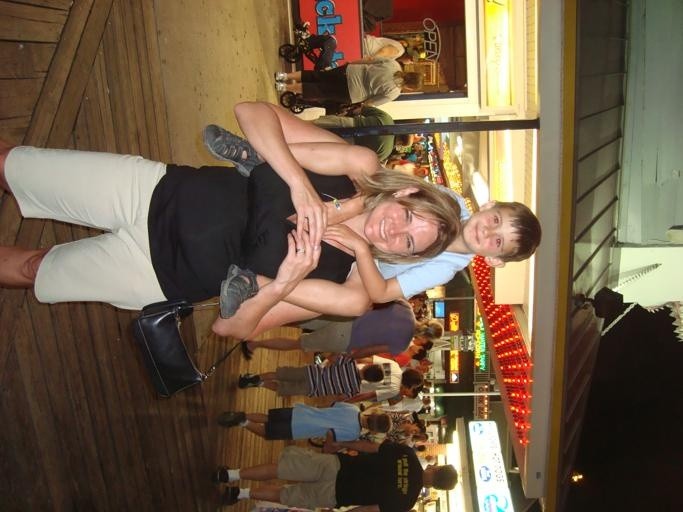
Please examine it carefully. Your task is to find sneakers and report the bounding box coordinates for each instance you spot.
[274,70,287,92]
[216,341,260,506]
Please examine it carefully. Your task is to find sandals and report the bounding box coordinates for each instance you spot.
[203,123,260,179]
[219,263,259,319]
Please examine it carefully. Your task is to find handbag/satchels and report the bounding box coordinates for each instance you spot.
[132,295,253,400]
